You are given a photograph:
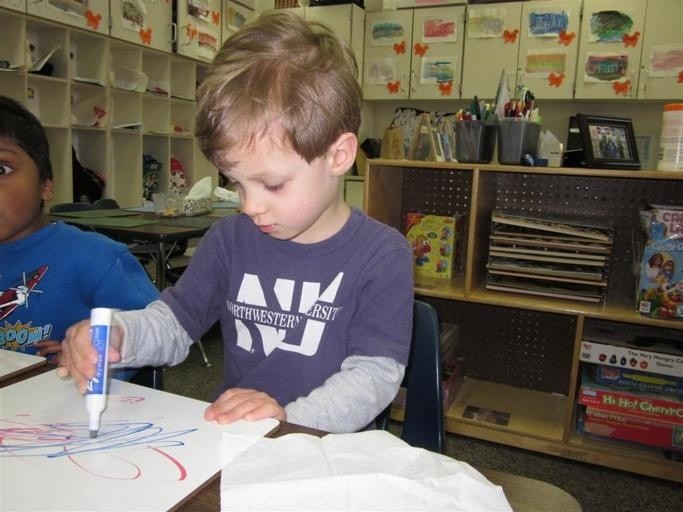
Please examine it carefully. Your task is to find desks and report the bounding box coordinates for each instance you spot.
[48,188,239,292]
[0,356,584,512]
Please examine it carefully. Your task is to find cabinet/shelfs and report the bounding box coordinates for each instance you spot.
[0,0,255,203]
[272,1,682,210]
[363,158,682,485]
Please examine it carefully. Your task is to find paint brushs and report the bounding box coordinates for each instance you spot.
[83,307,113,438]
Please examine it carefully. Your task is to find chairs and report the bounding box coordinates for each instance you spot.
[94,196,119,211]
[51,202,95,216]
[366,299,446,457]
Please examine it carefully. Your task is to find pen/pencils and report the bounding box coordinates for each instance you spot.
[463,107,472,120]
[473,94,482,120]
[504,89,536,118]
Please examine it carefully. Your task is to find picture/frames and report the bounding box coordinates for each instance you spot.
[578,115,642,169]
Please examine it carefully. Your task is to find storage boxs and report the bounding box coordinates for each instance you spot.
[453,117,497,164]
[497,116,541,165]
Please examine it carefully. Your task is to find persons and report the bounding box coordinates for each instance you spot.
[591,126,629,160]
[60,10,414,432]
[0,95,161,381]
